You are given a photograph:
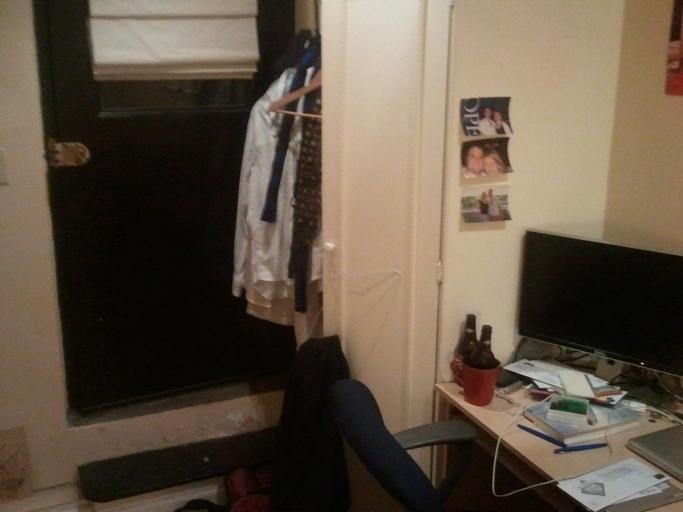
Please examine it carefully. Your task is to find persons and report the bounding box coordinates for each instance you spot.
[492,110,511,136]
[477,190,490,219]
[460,143,489,179]
[485,188,504,221]
[477,105,497,135]
[481,145,512,175]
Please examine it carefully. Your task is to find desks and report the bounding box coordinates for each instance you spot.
[432,378,682,510]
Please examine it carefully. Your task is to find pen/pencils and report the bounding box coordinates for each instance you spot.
[518,424,568,448]
[554,444,607,454]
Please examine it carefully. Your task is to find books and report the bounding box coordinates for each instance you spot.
[522,399,647,448]
[545,393,591,428]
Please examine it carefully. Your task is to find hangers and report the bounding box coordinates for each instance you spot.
[264,70,323,121]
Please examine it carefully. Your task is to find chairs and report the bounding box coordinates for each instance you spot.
[328,377,555,511]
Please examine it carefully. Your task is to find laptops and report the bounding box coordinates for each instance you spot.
[627,424,683,483]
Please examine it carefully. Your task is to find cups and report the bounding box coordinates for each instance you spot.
[451,360,500,406]
[450,353,464,387]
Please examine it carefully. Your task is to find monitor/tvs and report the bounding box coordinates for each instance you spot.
[518,228,683,384]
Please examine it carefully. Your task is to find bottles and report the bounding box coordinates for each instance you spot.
[469,326,496,368]
[458,313,478,359]
